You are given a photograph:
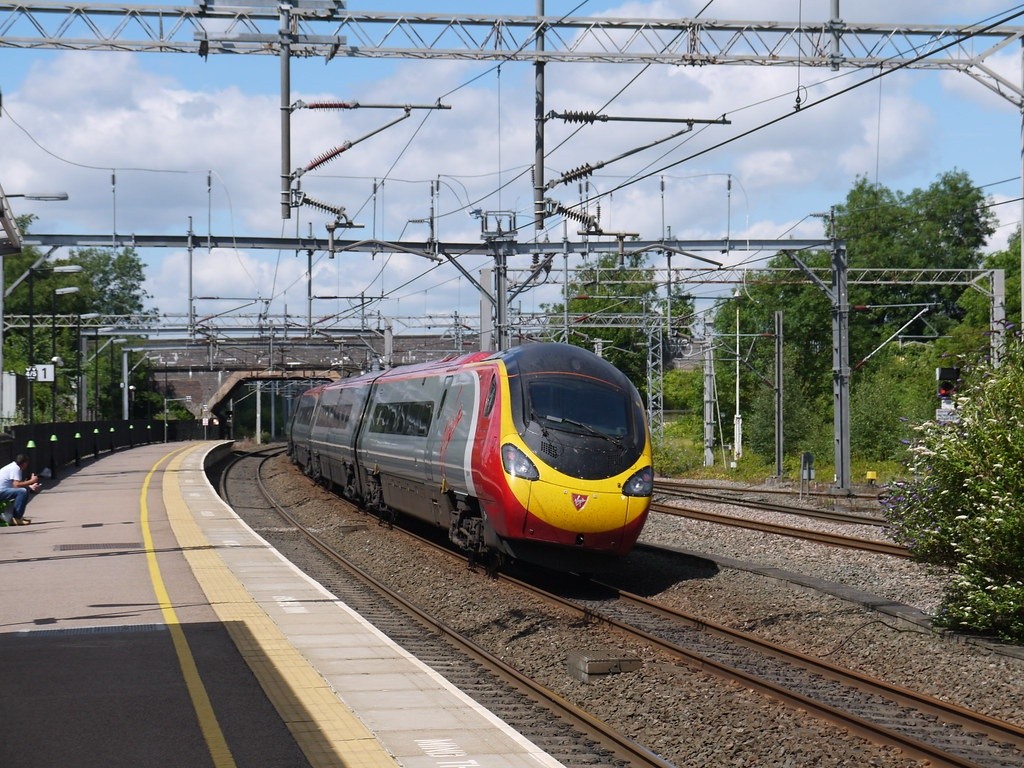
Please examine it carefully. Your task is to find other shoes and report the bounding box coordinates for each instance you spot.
[9,517,31,526]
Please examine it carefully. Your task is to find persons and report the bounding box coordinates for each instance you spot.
[0,452,42,526]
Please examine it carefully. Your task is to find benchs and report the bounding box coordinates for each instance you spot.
[0,495,21,526]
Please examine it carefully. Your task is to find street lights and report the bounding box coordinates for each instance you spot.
[94,327,114,421]
[109,338,128,420]
[29,265,84,424]
[76,312,100,422]
[52,287,80,422]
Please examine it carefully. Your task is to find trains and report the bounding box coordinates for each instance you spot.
[283,343,654,565]
